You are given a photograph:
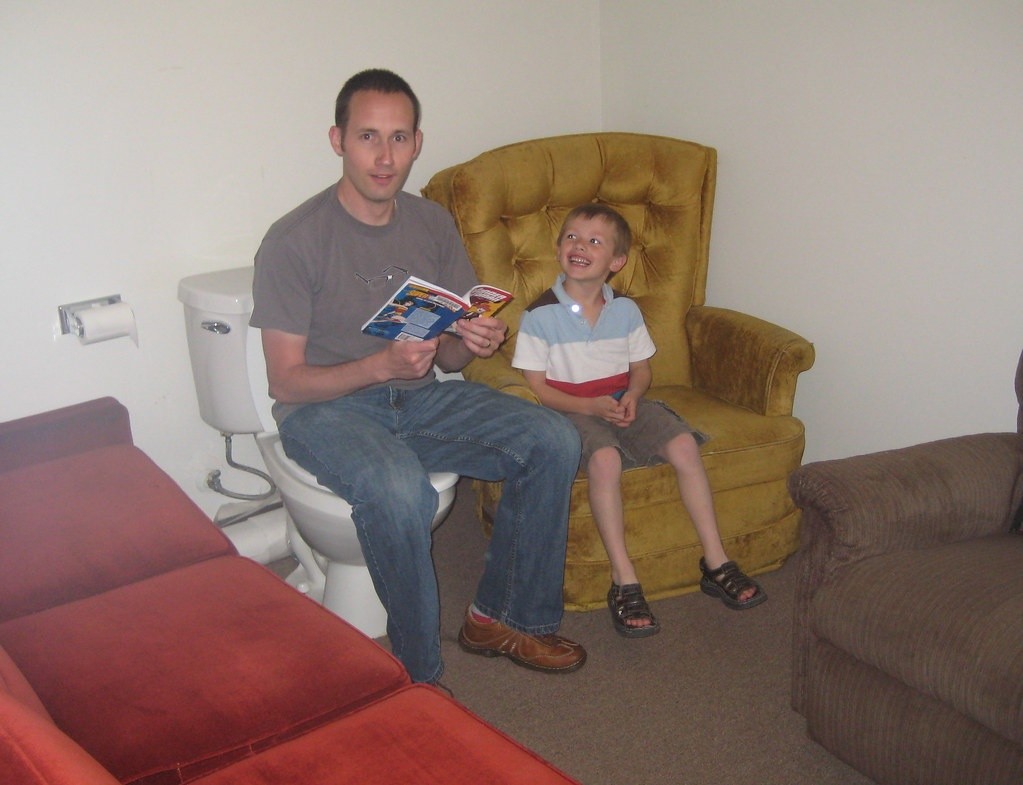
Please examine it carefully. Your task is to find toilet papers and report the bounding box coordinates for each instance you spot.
[73,302,135,345]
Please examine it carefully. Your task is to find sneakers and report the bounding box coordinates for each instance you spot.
[433,683,453,700]
[458,603,587,674]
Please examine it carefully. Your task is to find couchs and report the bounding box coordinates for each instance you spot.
[788,347,1023,785]
[0,397,577,785]
[417,131,815,610]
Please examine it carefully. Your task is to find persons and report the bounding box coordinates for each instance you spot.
[248,67,587,700]
[511,202,768,637]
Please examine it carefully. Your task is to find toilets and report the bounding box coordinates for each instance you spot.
[179,265,464,638]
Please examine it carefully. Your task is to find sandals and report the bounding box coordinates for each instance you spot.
[607,582,660,638]
[700,557,768,609]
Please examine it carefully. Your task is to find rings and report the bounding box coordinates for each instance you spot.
[487,343,490,347]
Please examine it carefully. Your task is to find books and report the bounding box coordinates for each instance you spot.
[359,276,512,341]
